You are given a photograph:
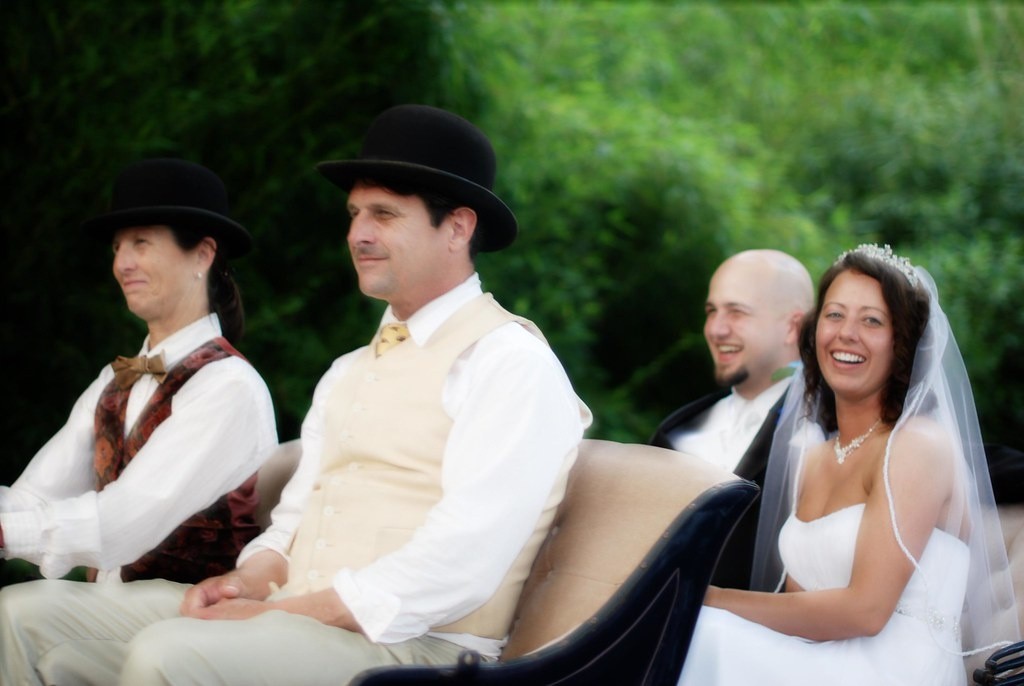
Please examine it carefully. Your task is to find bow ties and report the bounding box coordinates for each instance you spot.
[111,355,169,385]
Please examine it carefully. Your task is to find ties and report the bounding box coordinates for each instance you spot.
[375,324,410,359]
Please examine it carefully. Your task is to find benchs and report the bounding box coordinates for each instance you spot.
[963,440,1024,686]
[254,437,762,686]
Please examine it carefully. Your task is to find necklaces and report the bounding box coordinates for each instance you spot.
[832,415,883,464]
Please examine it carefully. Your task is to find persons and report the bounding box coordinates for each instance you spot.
[678,252,967,685]
[649,248,834,595]
[0,163,285,684]
[0,105,583,686]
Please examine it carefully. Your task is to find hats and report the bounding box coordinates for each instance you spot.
[315,105,518,253]
[58,161,254,266]
